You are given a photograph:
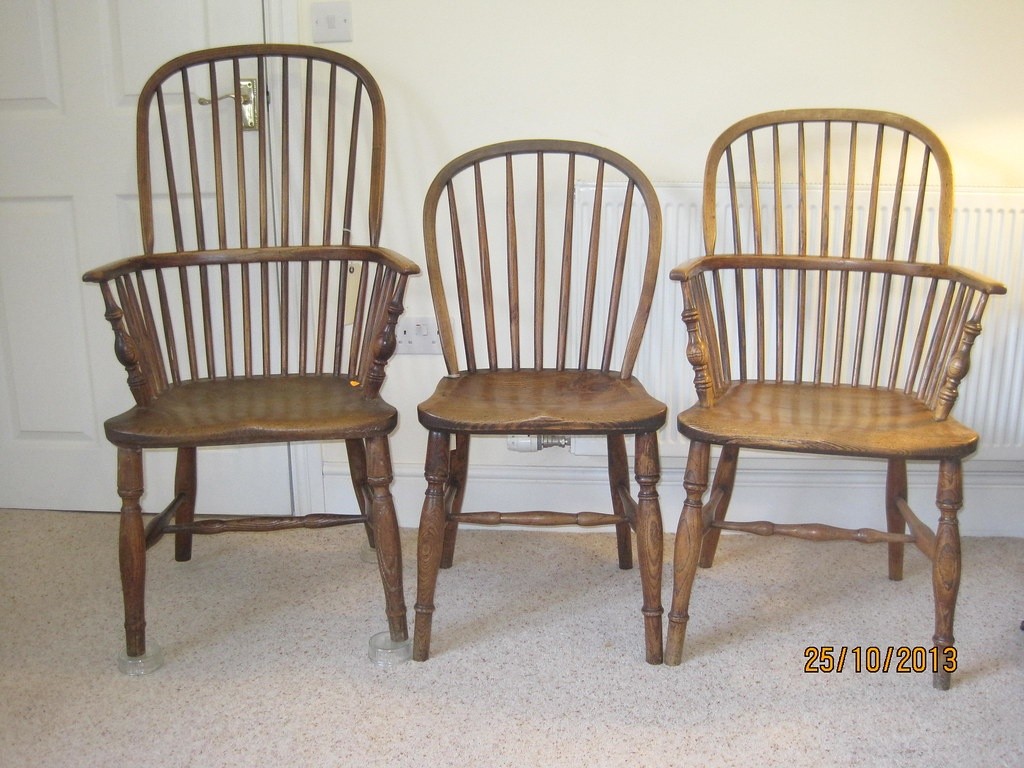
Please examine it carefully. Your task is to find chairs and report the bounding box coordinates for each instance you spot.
[664,107,1008,691]
[81,43,420,659]
[411,138,667,666]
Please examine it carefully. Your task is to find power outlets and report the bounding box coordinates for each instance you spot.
[393,315,454,355]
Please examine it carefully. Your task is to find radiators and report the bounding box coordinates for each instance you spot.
[507,178,1024,463]
